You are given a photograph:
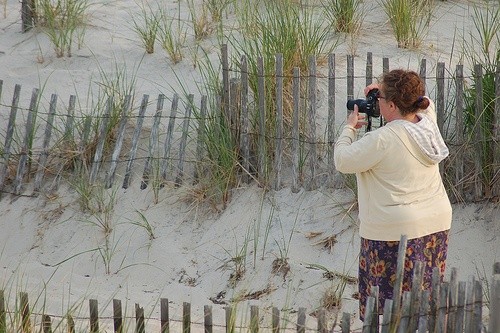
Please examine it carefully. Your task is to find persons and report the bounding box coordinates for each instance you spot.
[334,69,452,323]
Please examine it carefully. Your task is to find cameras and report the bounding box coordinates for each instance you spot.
[347,88,381,117]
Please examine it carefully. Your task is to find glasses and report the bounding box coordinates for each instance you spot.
[374,89,387,101]
